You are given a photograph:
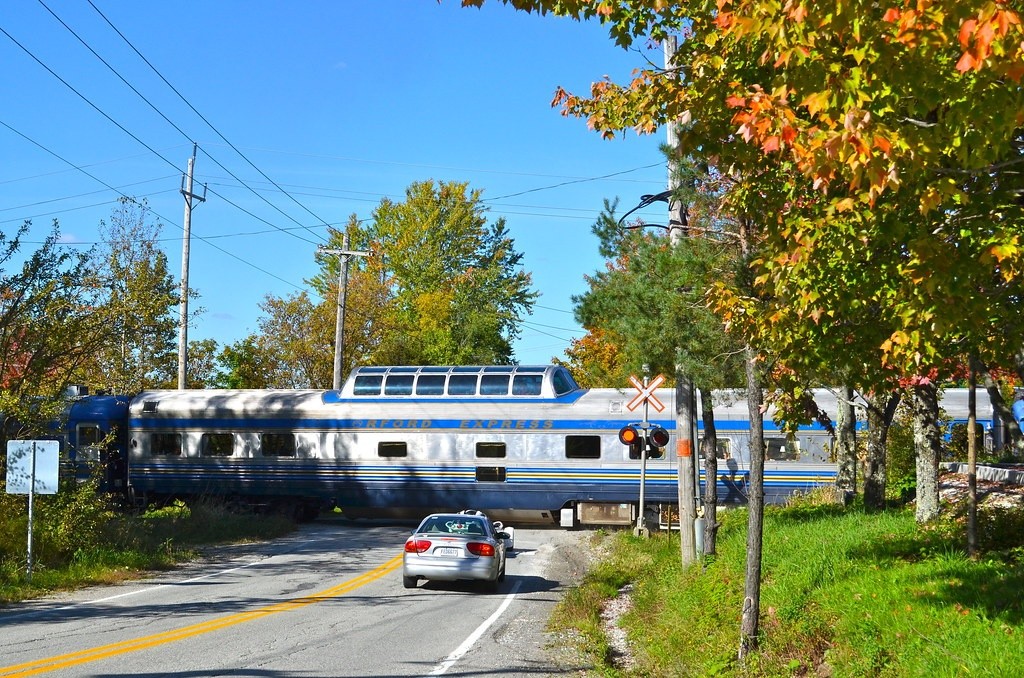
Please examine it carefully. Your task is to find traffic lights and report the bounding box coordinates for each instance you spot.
[650,428,669,448]
[619,426,639,445]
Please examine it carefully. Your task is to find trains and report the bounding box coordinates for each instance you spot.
[0,364,1024,529]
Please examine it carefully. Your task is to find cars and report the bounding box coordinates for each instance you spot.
[402,513,510,593]
